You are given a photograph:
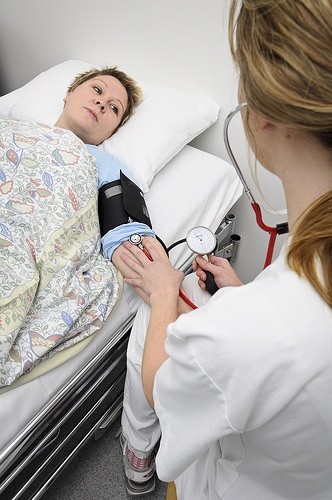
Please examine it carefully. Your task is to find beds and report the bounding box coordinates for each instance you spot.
[0,60,245,500]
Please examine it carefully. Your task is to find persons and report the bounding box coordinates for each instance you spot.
[0,64,194,386]
[113,1,332,500]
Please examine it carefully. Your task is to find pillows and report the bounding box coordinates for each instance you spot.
[0,59,220,195]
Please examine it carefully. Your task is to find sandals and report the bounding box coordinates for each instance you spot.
[118,425,156,494]
